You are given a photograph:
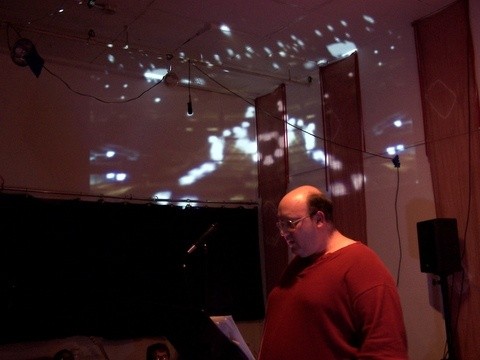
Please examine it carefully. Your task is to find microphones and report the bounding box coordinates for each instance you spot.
[187,222,218,255]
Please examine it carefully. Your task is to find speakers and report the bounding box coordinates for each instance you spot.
[417,218,462,273]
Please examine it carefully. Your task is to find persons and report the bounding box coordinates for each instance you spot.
[55,349,74,360]
[258,186,410,360]
[146,343,170,360]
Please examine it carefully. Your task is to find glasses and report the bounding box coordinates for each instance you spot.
[276,216,309,232]
